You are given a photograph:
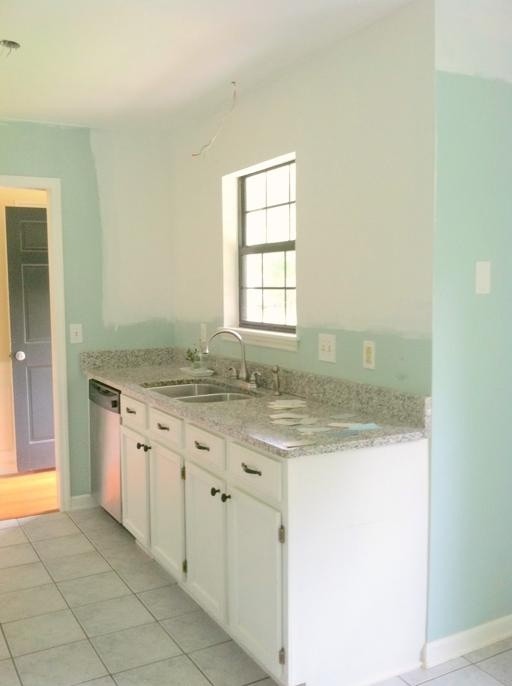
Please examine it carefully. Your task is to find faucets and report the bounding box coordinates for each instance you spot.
[203,327,250,381]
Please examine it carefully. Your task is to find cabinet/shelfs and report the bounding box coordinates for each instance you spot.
[185,423,427,686]
[117,395,185,592]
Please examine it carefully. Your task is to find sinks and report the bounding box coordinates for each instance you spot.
[173,392,264,405]
[138,376,231,397]
